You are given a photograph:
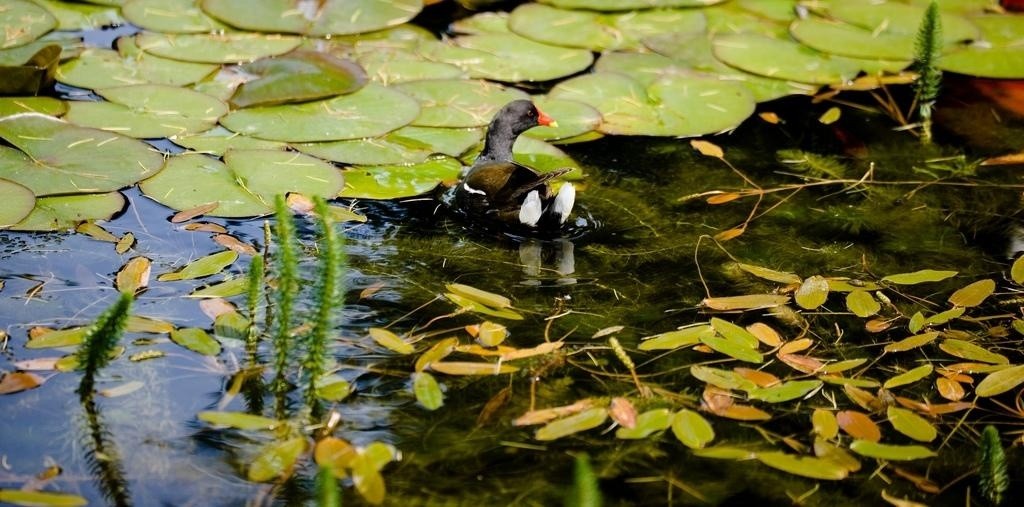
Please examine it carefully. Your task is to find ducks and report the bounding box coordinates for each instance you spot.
[449,99,578,234]
